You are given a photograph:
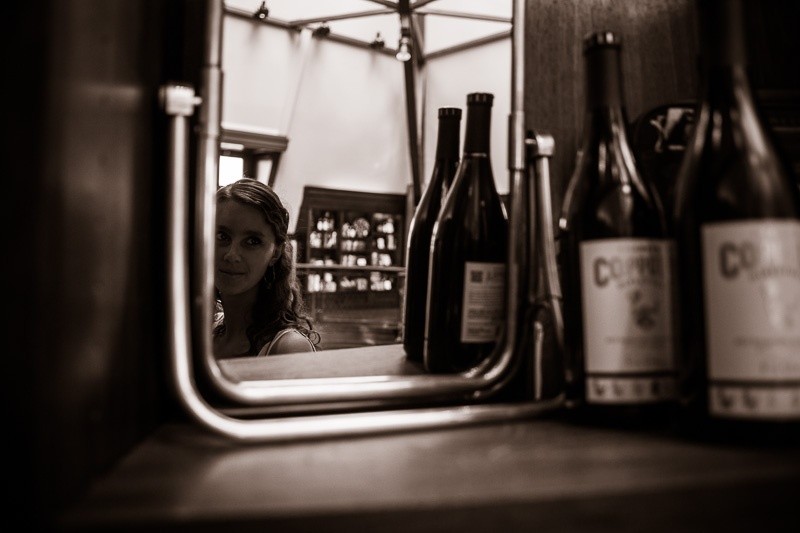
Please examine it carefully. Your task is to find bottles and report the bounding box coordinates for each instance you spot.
[673,1,800,450]
[560,28,680,429]
[404,107,461,360]
[423,94,509,375]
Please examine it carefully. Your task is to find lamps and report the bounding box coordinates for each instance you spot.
[395,32,411,61]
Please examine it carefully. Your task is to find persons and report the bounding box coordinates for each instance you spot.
[209,178,320,361]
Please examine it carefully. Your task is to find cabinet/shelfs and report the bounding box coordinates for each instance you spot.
[297,186,405,307]
